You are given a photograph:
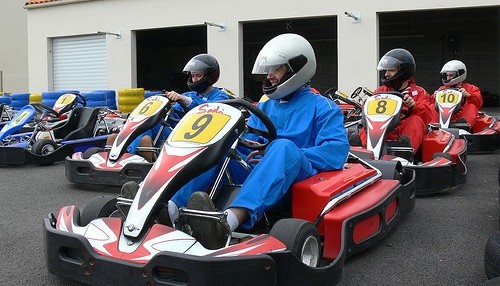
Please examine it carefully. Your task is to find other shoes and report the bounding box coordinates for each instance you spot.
[185,190,233,252]
[140,135,155,161]
[395,137,411,160]
[119,180,141,206]
[348,133,362,148]
[106,132,116,146]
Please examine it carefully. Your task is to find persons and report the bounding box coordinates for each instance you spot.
[349,48,432,164]
[120,32,350,249]
[430,60,483,133]
[107,54,230,164]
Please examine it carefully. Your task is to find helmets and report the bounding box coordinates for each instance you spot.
[439,59,467,87]
[180,52,221,92]
[251,32,317,101]
[377,47,417,90]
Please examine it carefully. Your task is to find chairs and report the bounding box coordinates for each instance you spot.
[51,106,101,143]
